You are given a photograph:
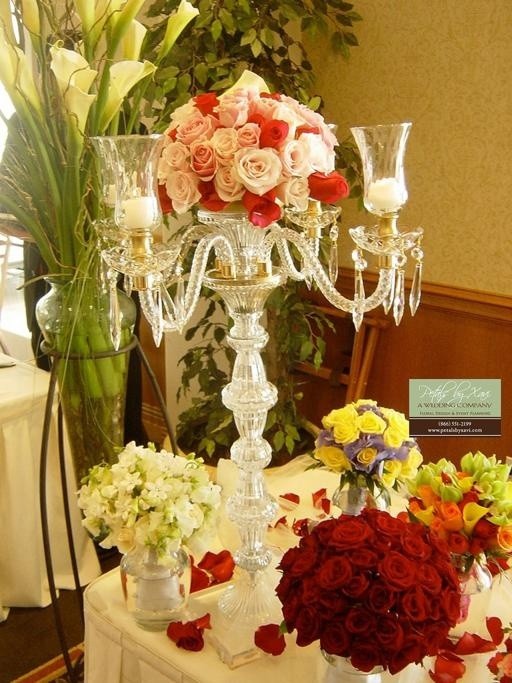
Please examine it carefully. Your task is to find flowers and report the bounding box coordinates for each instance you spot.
[0,0,210,469]
[68,429,226,566]
[138,69,356,228]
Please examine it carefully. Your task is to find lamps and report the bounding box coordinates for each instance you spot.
[84,112,435,676]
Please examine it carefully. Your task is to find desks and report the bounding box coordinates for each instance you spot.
[2,345,107,629]
[78,444,511,681]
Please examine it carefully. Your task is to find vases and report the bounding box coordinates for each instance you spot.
[28,268,145,546]
[110,536,196,636]
